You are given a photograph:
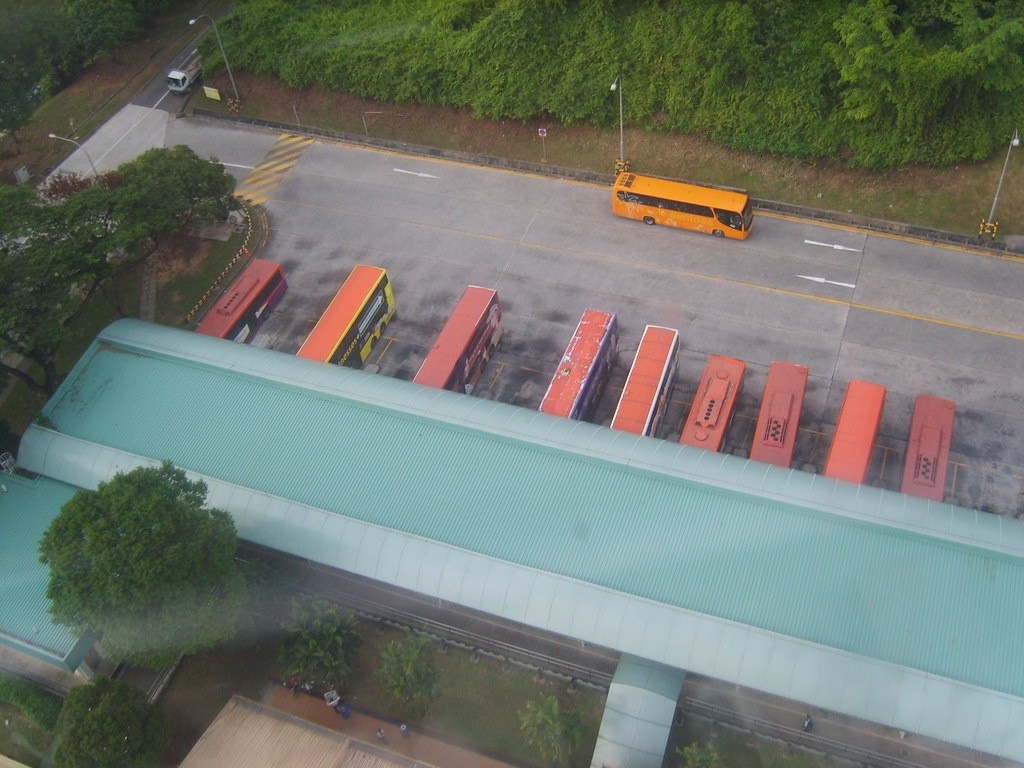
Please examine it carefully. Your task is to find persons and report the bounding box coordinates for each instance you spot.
[376,728,388,745]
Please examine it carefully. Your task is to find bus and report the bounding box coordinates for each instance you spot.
[609,324,681,437]
[900,394,957,503]
[612,171,755,242]
[749,361,810,469]
[295,264,397,369]
[822,380,887,485]
[410,284,505,395]
[535,308,619,422]
[194,258,288,345]
[676,353,747,454]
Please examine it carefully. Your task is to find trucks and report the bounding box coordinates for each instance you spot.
[165,49,202,96]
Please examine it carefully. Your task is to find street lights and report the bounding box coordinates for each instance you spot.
[610,74,624,165]
[986,127,1021,232]
[48,134,98,177]
[189,14,241,103]
